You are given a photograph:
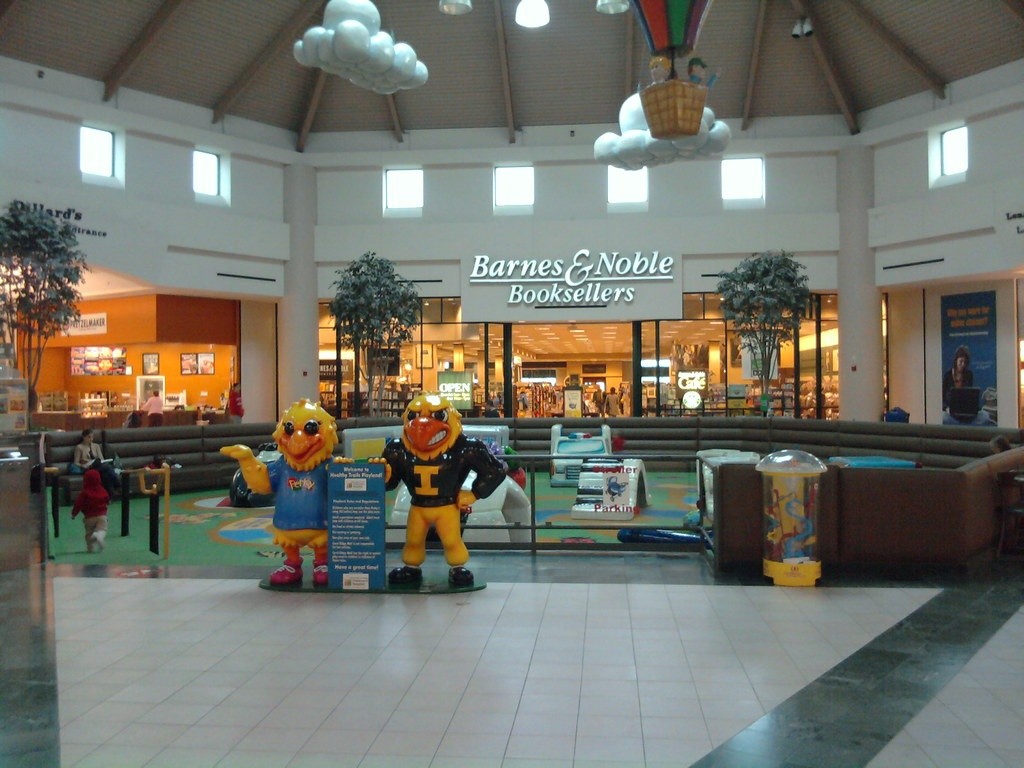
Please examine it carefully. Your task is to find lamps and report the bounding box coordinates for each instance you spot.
[594,0,630,15]
[438,0,473,16]
[792,16,814,40]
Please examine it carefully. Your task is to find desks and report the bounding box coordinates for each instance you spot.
[991,474,1024,488]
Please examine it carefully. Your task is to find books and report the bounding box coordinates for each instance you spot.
[321,391,421,418]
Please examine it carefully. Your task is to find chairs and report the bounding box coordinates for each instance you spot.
[995,470,1024,559]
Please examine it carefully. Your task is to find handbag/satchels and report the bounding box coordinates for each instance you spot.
[604,395,609,414]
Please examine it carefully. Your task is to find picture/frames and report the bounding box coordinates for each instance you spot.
[415,343,434,369]
[142,352,160,375]
[197,353,215,375]
[180,353,198,376]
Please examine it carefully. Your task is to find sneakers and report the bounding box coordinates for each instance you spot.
[86,533,105,553]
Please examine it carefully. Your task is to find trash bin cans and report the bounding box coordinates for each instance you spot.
[886,407,909,423]
[1,434,62,768]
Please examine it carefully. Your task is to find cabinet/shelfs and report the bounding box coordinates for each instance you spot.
[37,390,68,411]
[346,390,413,418]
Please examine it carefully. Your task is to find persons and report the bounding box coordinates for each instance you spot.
[70,470,111,554]
[689,57,720,91]
[605,386,620,417]
[122,412,143,429]
[141,391,163,427]
[647,57,672,85]
[229,383,244,424]
[942,345,973,411]
[75,428,133,499]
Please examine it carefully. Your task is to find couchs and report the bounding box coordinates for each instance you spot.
[332,415,1024,582]
[44,422,277,504]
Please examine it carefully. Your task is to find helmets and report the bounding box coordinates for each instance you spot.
[953,344,969,359]
